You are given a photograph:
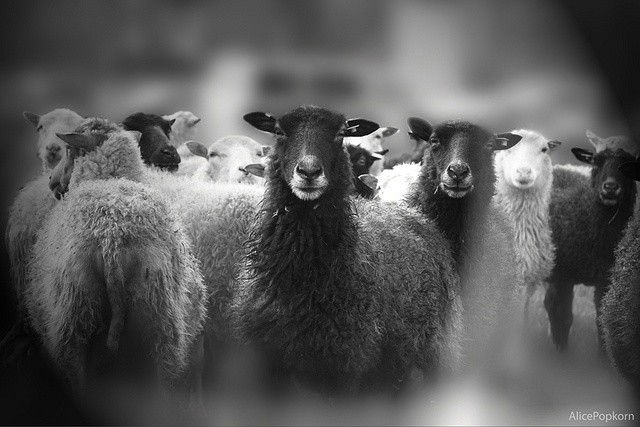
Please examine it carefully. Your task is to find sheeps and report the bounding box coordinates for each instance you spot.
[403,115,520,284]
[344,115,398,175]
[24,117,209,381]
[20,105,86,170]
[239,104,464,414]
[372,127,557,388]
[544,144,639,383]
[120,109,182,175]
[187,135,277,190]
